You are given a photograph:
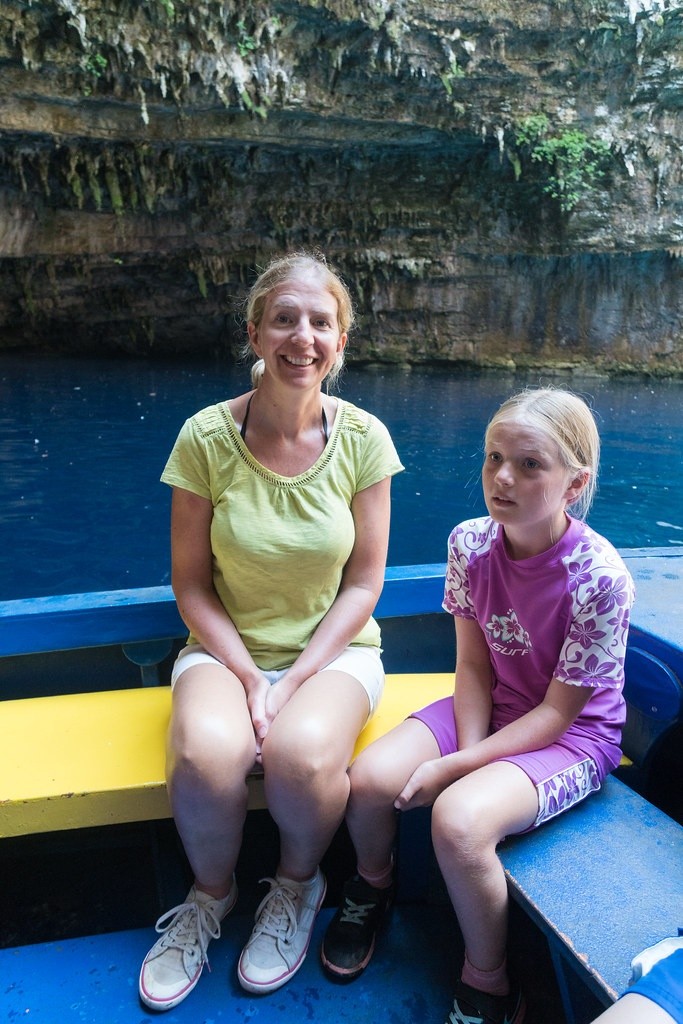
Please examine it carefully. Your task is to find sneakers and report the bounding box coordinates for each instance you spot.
[138,869,238,1011]
[321,875,398,977]
[445,977,525,1024]
[237,865,329,994]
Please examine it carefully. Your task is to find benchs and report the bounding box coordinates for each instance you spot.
[0,672,683,1023]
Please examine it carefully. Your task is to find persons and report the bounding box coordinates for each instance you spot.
[318,389,636,1024]
[137,252,405,1010]
